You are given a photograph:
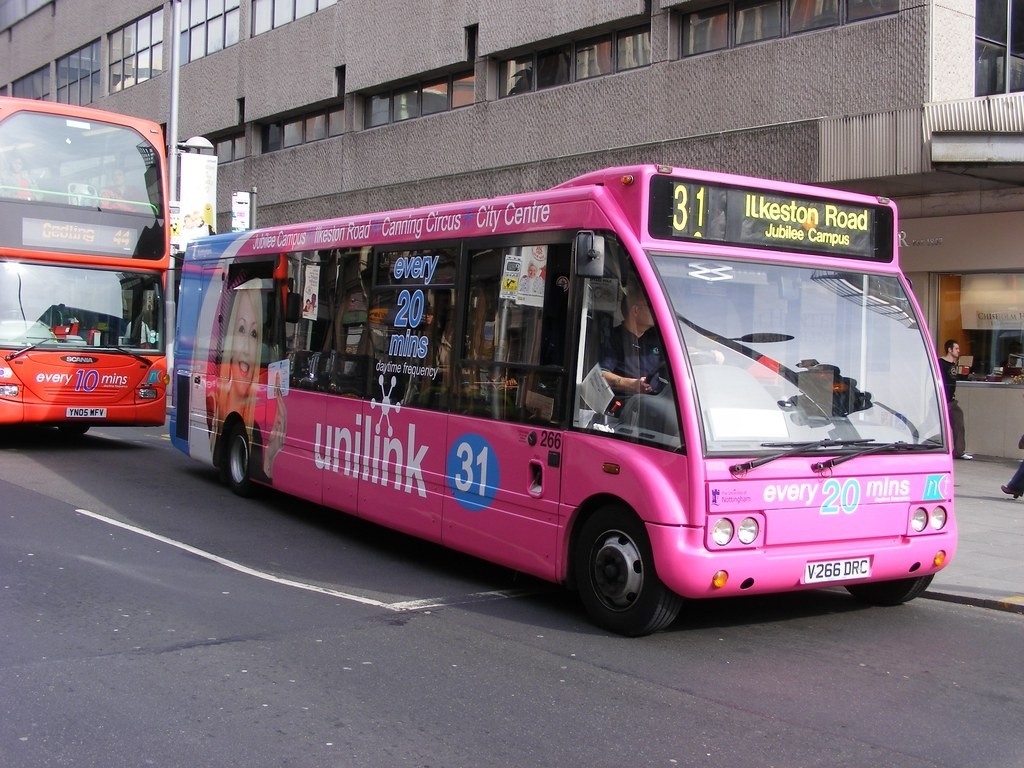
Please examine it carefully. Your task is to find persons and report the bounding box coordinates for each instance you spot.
[304,294,316,312]
[209,290,287,483]
[100,170,137,211]
[1001,459,1024,498]
[597,292,725,417]
[522,265,541,293]
[0,156,43,200]
[938,340,973,459]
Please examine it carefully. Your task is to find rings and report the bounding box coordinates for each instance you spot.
[278,421,281,425]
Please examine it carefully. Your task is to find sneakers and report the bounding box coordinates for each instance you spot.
[961,454,973,460]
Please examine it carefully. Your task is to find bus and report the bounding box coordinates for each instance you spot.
[167,162,959,636]
[0,93,176,436]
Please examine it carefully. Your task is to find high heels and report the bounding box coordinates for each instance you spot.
[1001,485,1020,499]
